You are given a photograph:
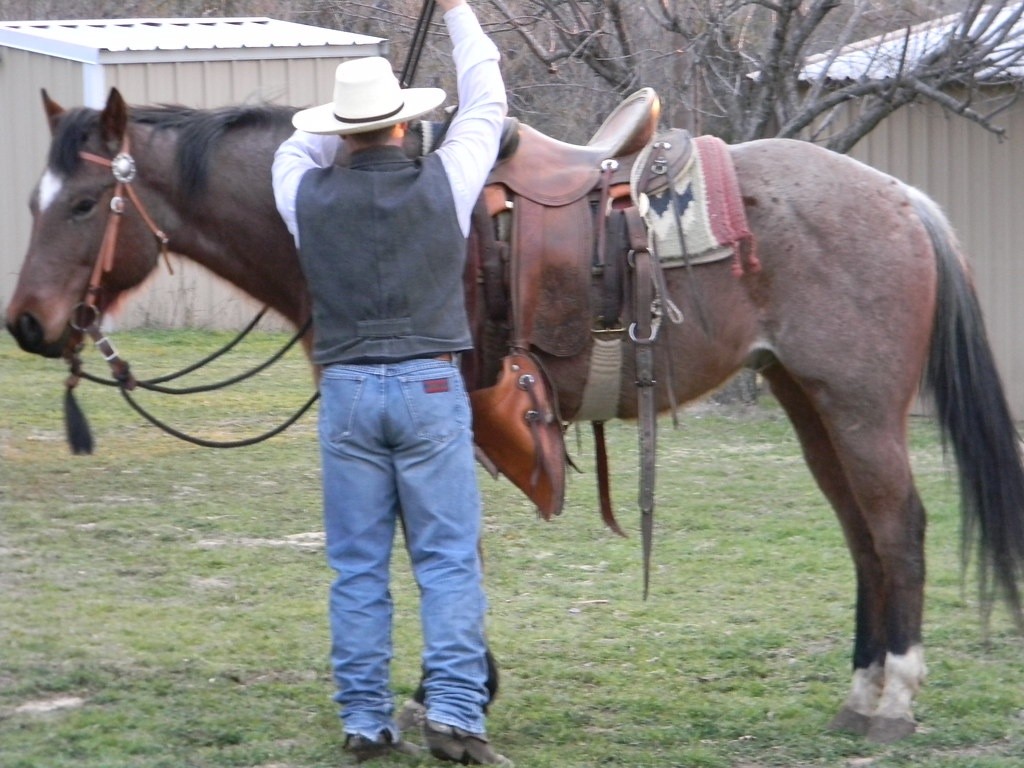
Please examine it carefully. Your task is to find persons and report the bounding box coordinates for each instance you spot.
[270,1,515,768]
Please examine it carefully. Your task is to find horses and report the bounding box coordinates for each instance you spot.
[0,79,1024,744]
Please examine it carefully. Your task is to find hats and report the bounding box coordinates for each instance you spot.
[290,56,447,136]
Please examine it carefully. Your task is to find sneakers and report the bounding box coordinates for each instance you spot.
[424,720,513,765]
[341,731,405,759]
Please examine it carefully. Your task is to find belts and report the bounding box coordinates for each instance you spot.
[436,349,465,366]
